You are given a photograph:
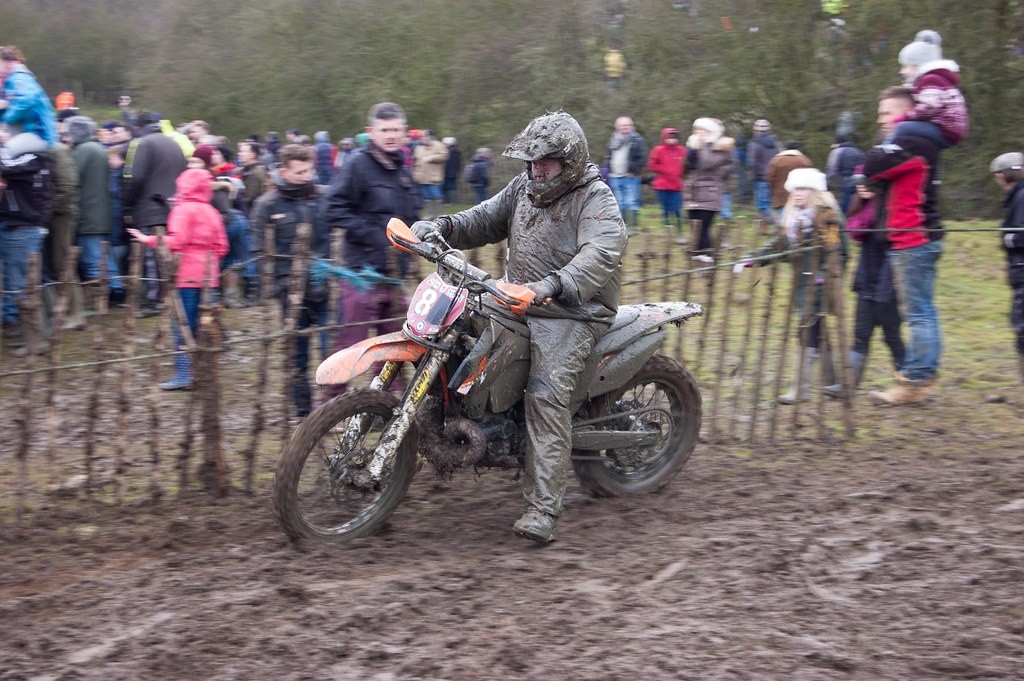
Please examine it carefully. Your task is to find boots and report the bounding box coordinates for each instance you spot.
[822,350,865,397]
[158,351,197,391]
[777,344,814,404]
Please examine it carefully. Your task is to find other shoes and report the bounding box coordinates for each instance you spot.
[867,372,935,406]
[1,286,224,356]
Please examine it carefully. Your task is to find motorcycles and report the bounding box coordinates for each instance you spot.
[272,216,704,555]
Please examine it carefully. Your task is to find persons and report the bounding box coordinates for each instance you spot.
[990,152,1024,358]
[127,169,231,390]
[679,116,741,264]
[719,118,787,236]
[408,109,629,547]
[761,29,970,407]
[0,42,496,358]
[733,166,849,405]
[317,101,425,433]
[605,117,650,227]
[646,127,690,236]
[248,143,336,428]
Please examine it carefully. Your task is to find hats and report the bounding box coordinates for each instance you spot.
[67,116,97,144]
[988,152,1023,174]
[691,117,725,137]
[195,146,216,166]
[441,136,457,146]
[752,119,771,135]
[897,29,944,66]
[784,168,828,193]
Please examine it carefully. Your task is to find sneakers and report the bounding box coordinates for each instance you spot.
[700,256,715,263]
[692,254,708,261]
[513,509,559,545]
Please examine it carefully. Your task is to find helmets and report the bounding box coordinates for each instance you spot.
[501,109,589,208]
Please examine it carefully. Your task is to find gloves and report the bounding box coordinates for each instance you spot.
[409,217,448,241]
[522,276,560,307]
